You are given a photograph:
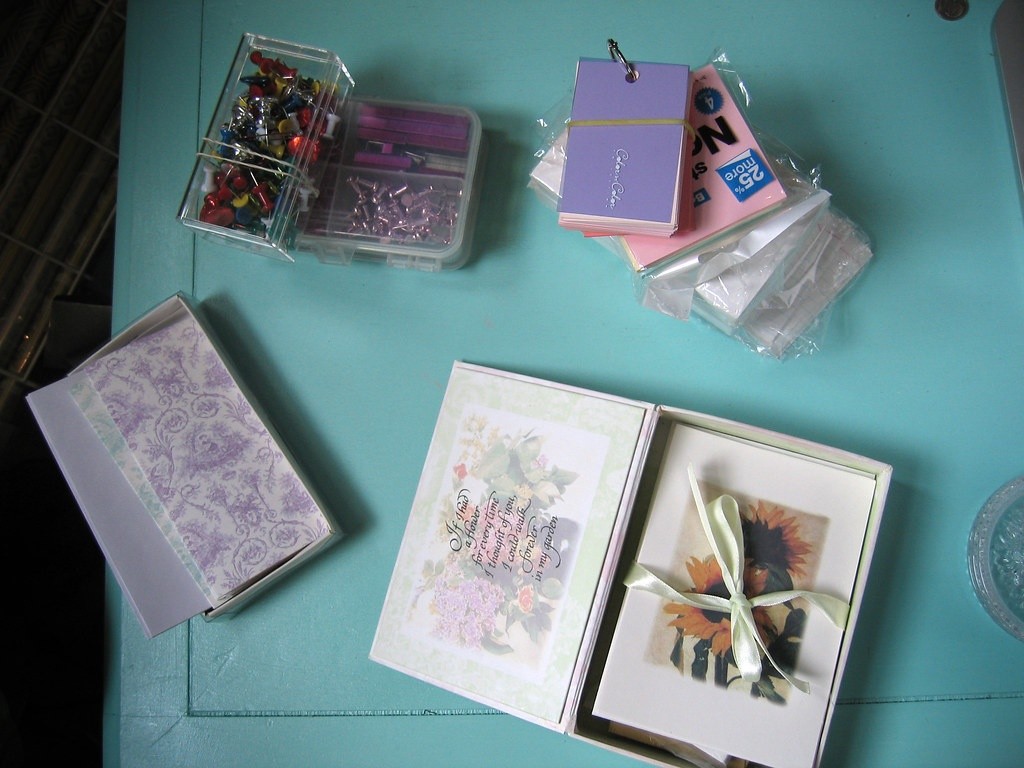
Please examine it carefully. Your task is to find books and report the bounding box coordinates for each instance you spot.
[528,40,874,361]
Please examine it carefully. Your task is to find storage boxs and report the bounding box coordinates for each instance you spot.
[174,31,483,273]
[24,289,345,639]
[367,359,893,768]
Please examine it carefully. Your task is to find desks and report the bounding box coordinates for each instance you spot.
[93,0,1023,768]
